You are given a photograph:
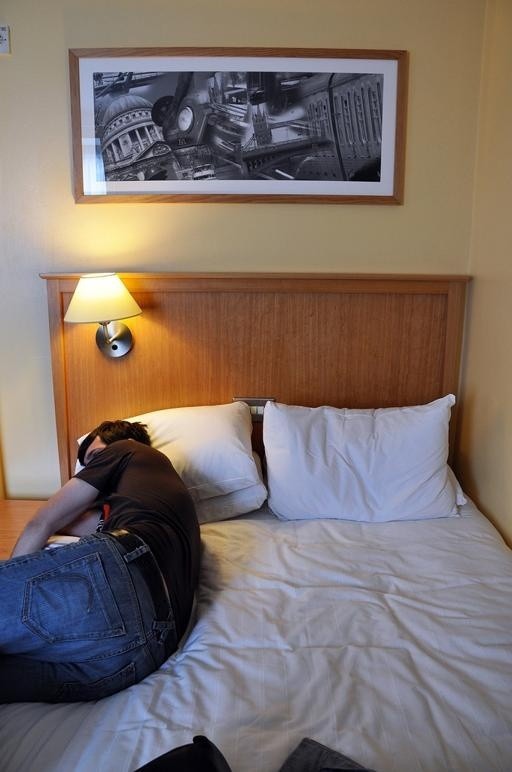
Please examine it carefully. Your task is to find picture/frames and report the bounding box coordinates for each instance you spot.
[68,45,411,206]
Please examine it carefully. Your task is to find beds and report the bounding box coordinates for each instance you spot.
[0,270,512,772]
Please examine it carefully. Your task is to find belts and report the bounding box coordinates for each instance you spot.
[100,526,179,660]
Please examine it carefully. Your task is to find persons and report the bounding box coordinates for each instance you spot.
[1,418,206,706]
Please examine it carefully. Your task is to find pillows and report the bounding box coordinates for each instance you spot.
[447,463,470,508]
[261,392,461,525]
[69,450,270,525]
[72,401,261,511]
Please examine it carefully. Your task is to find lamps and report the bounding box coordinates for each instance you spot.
[63,273,146,359]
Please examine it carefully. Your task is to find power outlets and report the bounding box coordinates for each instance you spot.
[0,25,12,54]
